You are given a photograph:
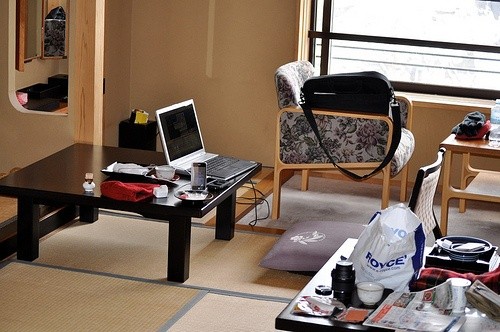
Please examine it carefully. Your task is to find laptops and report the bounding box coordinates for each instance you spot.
[155,98,257,180]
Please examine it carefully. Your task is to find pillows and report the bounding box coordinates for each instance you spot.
[260,217,368,274]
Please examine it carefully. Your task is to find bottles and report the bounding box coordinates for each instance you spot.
[488,98,500,147]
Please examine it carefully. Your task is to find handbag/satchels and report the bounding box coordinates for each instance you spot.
[298,70,402,181]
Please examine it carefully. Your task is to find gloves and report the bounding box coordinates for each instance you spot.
[452,110,485,137]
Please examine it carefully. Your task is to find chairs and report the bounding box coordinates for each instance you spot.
[273,60,416,219]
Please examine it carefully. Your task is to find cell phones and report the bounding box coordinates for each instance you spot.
[190,162,207,190]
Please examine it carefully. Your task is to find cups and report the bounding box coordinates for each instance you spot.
[446,278,471,312]
[155,166,175,179]
[357,282,384,305]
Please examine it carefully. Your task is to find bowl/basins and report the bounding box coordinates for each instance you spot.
[437,236,493,262]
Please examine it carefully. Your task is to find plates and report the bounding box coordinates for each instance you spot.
[101,160,156,179]
[175,191,213,201]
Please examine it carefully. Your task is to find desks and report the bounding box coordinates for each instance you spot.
[275,238,436,332]
[439,125,500,239]
[0,143,262,283]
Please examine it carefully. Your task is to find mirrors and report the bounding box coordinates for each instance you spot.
[17,0,44,72]
